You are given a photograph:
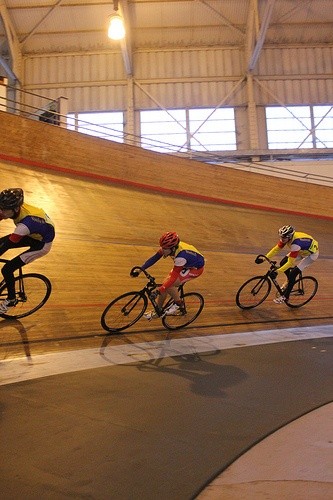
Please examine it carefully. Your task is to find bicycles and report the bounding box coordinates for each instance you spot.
[235,254,319,310]
[0,258,52,321]
[100,266,204,333]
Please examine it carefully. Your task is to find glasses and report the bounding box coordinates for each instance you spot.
[278,236,289,239]
[162,247,169,250]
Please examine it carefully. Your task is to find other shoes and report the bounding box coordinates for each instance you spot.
[280,286,289,292]
[273,295,288,304]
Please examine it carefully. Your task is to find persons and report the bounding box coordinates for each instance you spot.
[257,225,319,304]
[132,231,205,318]
[0,188,56,316]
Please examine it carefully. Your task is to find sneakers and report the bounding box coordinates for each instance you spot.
[0,298,17,314]
[165,300,185,315]
[143,307,165,318]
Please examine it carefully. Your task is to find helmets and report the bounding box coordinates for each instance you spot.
[159,232,180,248]
[0,188,24,210]
[278,225,295,237]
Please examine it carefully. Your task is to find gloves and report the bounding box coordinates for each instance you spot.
[254,259,263,264]
[149,292,159,301]
[131,271,141,277]
[270,272,278,280]
